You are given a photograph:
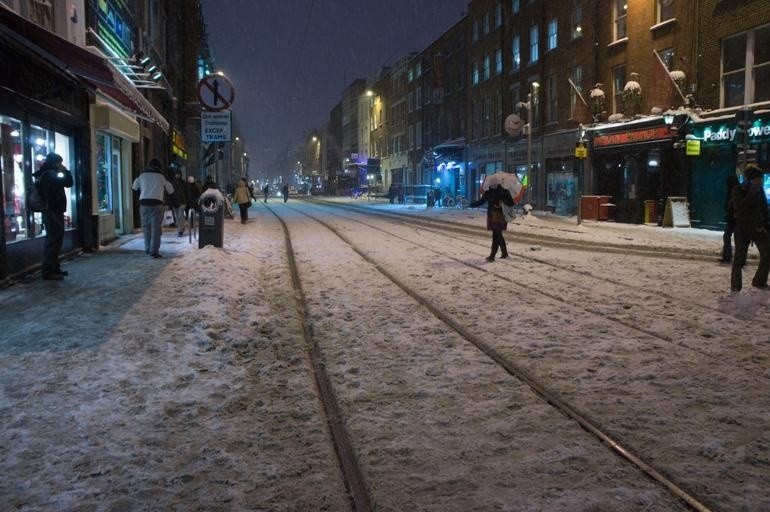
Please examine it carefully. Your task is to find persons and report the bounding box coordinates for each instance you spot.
[283,183,290,202]
[32,152,75,281]
[264,184,269,203]
[465,177,515,262]
[426,184,462,208]
[389,183,397,203]
[131,158,176,257]
[396,183,404,204]
[167,168,257,237]
[730,163,770,292]
[718,172,741,264]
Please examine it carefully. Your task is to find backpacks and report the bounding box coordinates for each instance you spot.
[27,170,52,212]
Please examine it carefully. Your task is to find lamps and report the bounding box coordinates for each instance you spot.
[109,51,164,84]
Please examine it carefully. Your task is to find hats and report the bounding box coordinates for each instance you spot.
[745,165,763,180]
[207,176,213,179]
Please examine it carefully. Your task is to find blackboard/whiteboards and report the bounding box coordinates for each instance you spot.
[671,197,690,226]
[663,197,673,226]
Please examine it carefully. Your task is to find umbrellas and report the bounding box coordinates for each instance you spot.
[482,170,522,199]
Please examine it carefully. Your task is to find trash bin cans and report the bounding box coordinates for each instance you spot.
[199,188,224,248]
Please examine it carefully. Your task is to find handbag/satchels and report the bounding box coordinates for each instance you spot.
[248,201,252,206]
[501,204,516,221]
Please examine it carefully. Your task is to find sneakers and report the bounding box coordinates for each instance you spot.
[42,270,67,280]
[487,255,495,260]
[731,284,742,290]
[716,259,730,263]
[501,252,508,258]
[751,280,770,291]
[241,217,248,223]
[144,251,162,259]
